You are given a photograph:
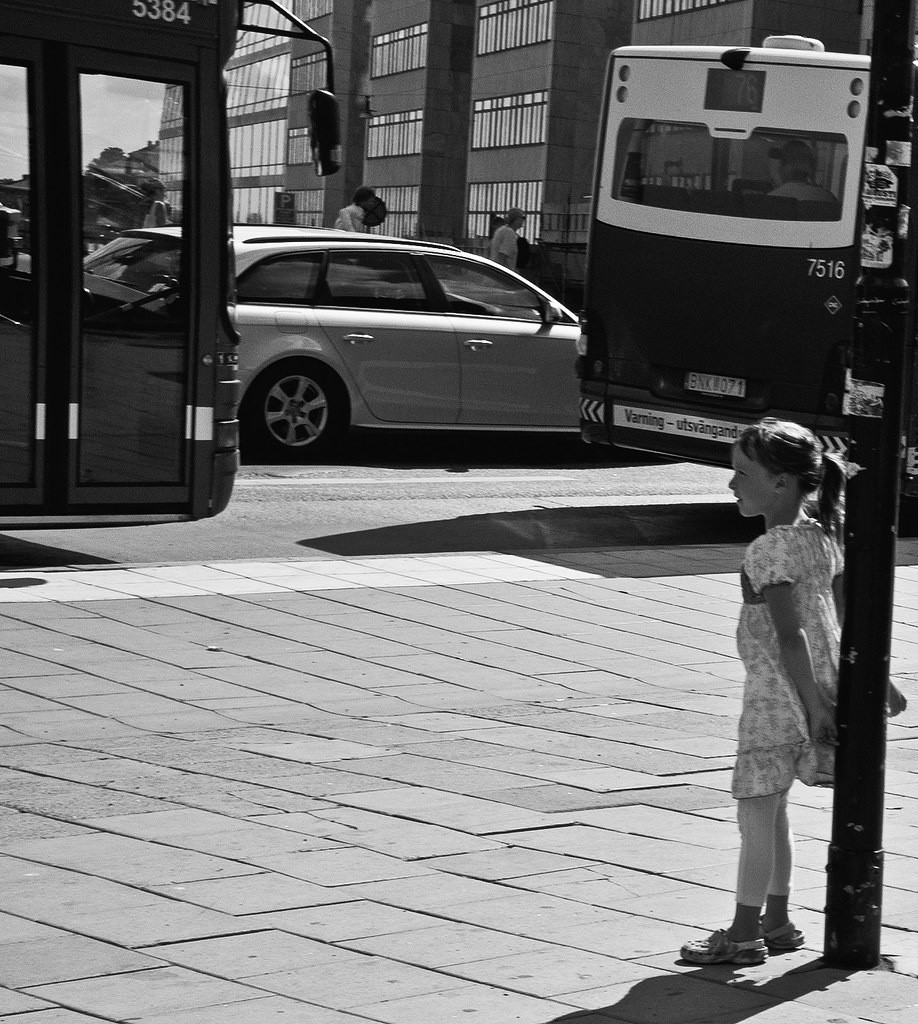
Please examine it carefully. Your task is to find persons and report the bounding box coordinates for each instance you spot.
[680,415,906,965]
[765,140,837,203]
[490,206,526,270]
[334,186,376,233]
[134,178,175,228]
[491,216,505,235]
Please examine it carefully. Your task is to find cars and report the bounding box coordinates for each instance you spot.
[83,223,599,463]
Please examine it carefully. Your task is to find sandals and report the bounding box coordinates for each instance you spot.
[758,913,806,950]
[681,928,768,964]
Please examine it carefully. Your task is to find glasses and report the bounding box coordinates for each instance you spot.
[519,215,526,220]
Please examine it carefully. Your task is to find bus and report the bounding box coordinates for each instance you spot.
[0,0,343,518]
[570,34,917,541]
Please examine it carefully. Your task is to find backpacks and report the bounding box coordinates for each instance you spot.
[516,236,530,269]
